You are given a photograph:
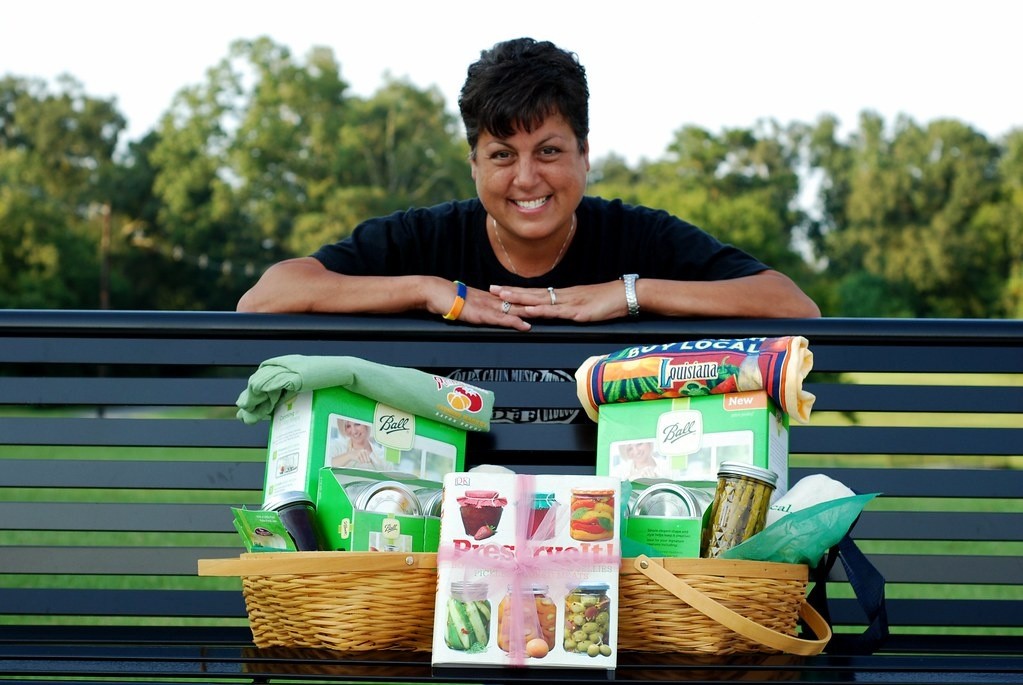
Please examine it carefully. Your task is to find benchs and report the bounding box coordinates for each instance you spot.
[0,305,1022,683]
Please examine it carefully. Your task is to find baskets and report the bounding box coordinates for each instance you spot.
[615,555,832,655]
[197,551,441,657]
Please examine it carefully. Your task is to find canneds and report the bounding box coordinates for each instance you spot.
[569,490,614,541]
[259,492,327,552]
[412,489,442,517]
[703,460,778,558]
[497,582,555,655]
[444,583,491,652]
[524,492,560,540]
[563,584,609,652]
[457,490,507,537]
[630,483,713,518]
[340,481,422,516]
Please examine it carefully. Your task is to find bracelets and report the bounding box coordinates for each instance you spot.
[442,279,467,319]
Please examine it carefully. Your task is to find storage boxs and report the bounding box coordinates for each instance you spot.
[205,544,446,658]
[620,554,834,660]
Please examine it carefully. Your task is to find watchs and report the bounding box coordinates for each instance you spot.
[615,272,641,315]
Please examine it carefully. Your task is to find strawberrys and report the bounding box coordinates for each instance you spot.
[474,521,495,540]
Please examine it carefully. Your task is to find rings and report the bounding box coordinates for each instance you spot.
[500,299,511,312]
[548,286,556,303]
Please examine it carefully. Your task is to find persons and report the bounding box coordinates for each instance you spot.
[233,38,824,470]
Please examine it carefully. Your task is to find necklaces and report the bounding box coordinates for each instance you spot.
[492,213,576,277]
